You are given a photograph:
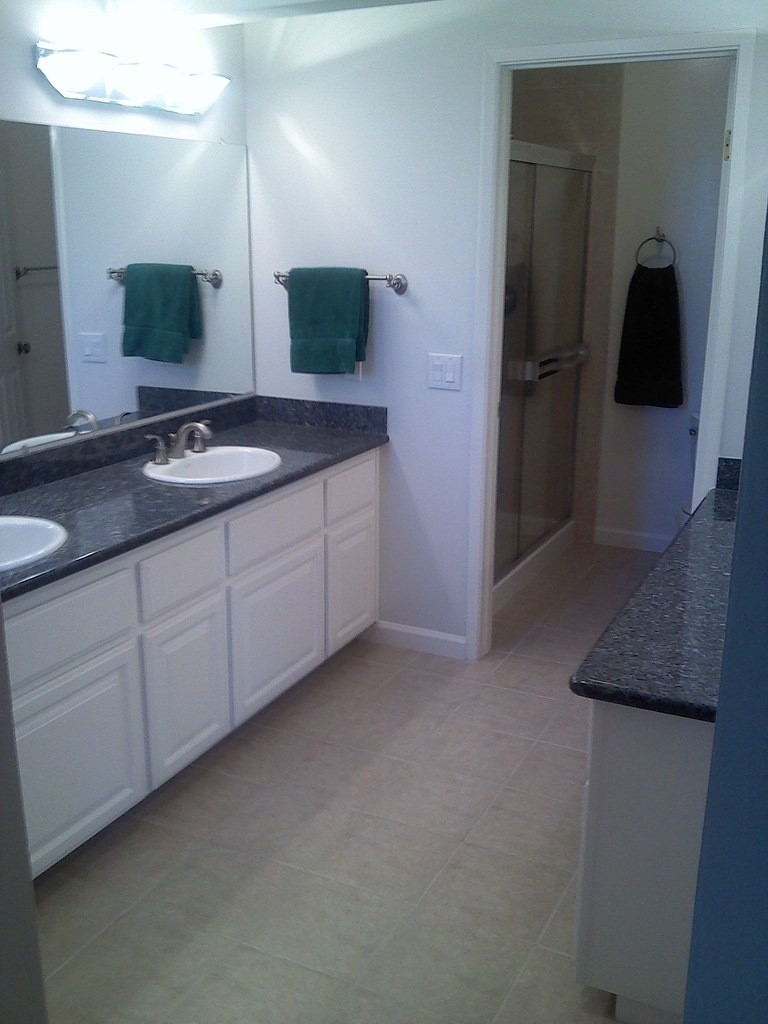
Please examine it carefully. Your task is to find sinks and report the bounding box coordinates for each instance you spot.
[2,431,97,454]
[0,506,70,579]
[142,443,286,488]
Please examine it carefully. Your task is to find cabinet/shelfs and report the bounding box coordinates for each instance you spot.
[2,445,382,885]
[571,699,716,1023]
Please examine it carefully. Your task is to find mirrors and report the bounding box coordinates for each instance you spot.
[0,118,258,491]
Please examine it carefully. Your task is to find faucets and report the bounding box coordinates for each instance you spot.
[163,421,212,460]
[65,411,103,432]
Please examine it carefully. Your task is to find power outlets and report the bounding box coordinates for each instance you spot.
[343,361,362,380]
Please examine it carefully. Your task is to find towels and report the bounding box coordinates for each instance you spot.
[609,261,688,410]
[121,261,206,366]
[286,266,372,375]
